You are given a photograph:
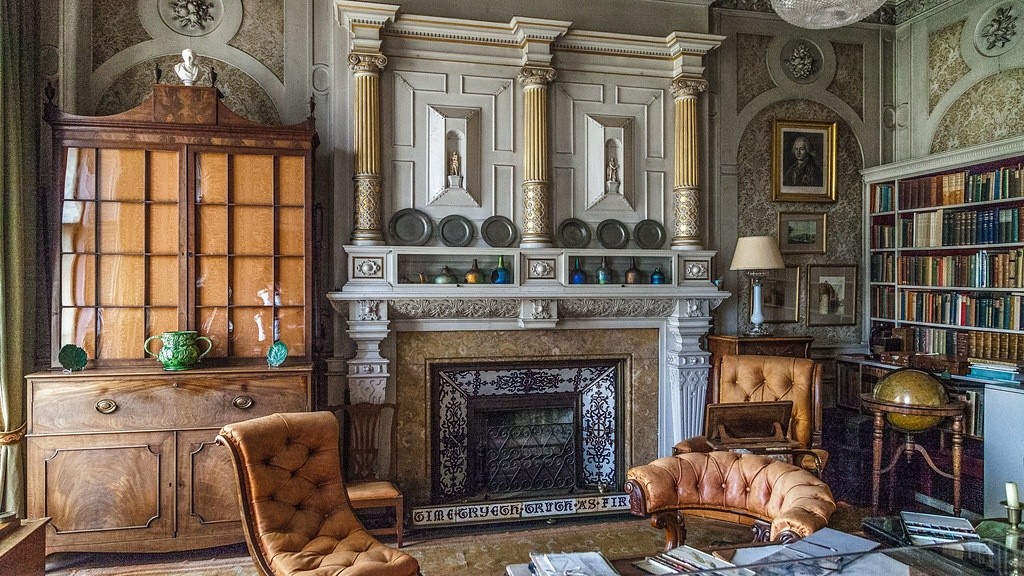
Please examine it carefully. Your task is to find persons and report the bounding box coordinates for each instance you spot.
[174,49,199,87]
[608,157,620,181]
[450,151,459,175]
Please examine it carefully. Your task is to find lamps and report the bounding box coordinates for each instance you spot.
[730,235,785,336]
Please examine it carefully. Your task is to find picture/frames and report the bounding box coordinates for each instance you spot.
[749,265,800,323]
[770,118,838,202]
[777,211,828,254]
[806,264,859,327]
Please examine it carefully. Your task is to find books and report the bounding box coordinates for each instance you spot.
[847,163,1024,440]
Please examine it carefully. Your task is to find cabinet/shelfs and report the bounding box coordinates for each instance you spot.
[24,63,316,556]
[834,136,1024,521]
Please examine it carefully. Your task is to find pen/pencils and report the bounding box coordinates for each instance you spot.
[802,539,838,552]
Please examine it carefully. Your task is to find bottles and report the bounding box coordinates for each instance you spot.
[569,257,586,284]
[625,256,642,284]
[465,259,485,284]
[596,256,612,284]
[418,271,428,284]
[491,257,511,284]
[434,266,457,284]
[651,268,665,284]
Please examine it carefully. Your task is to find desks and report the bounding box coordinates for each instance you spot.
[707,335,814,365]
[0,517,52,576]
[610,531,951,576]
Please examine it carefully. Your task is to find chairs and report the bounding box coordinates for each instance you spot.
[625,354,837,552]
[215,411,421,576]
[317,402,403,548]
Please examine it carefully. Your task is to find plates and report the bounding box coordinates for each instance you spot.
[633,220,666,249]
[389,209,433,246]
[267,342,288,365]
[558,218,591,248]
[596,219,628,249]
[481,215,516,248]
[58,344,87,371]
[437,215,474,247]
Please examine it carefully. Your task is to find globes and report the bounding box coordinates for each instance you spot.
[862,368,965,516]
